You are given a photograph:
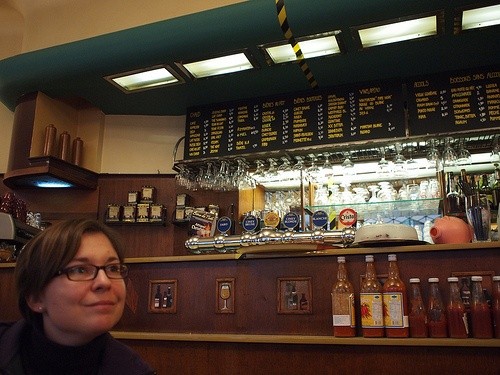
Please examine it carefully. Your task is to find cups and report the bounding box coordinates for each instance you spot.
[465,194,491,243]
[230,220,236,236]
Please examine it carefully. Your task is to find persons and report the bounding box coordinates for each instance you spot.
[0,218,155,375]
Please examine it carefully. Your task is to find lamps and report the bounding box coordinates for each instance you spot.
[102,0,500,95]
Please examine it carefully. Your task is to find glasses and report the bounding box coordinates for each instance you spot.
[52,263,128,281]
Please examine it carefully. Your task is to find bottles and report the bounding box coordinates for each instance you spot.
[360,255,385,337]
[383,254,409,338]
[72,137,83,166]
[43,124,57,157]
[470,276,492,339]
[427,278,447,338]
[255,212,261,235]
[447,277,469,339]
[59,131,71,162]
[408,278,428,338]
[443,169,468,224]
[467,169,500,223]
[492,276,500,339]
[331,257,355,337]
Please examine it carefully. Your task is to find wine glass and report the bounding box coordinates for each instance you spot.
[215,159,237,192]
[197,161,219,190]
[252,156,307,186]
[490,140,500,163]
[264,177,441,228]
[307,151,355,182]
[232,157,256,191]
[175,164,198,191]
[377,137,471,177]
